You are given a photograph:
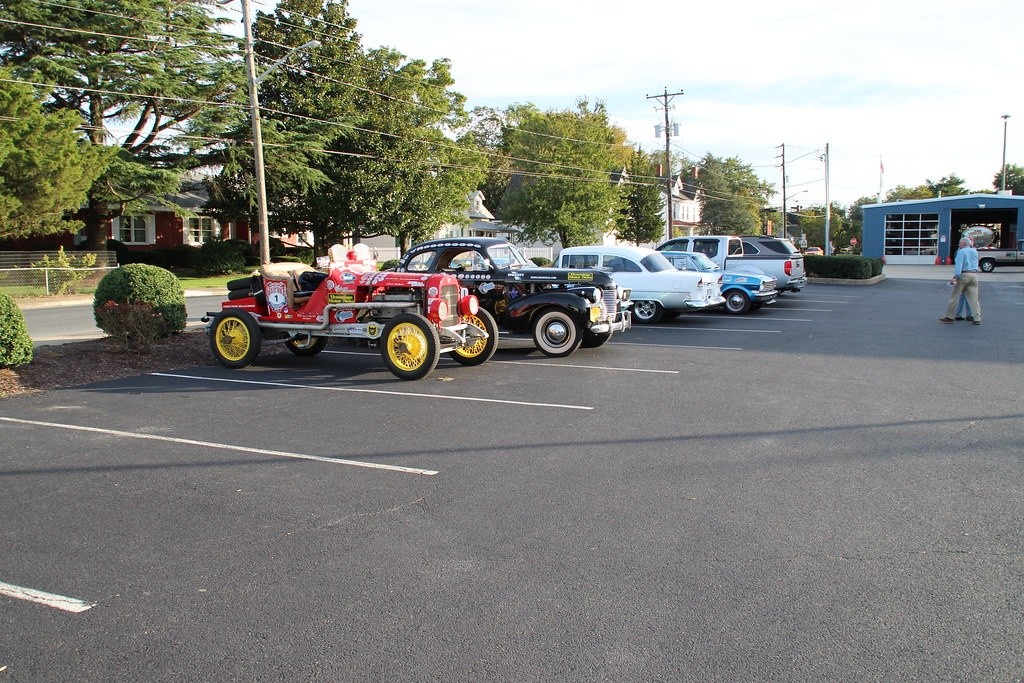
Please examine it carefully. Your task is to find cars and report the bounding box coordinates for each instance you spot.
[740,238,803,257]
[558,246,726,323]
[805,247,823,256]
[659,251,777,314]
[203,245,499,379]
[840,248,852,254]
[831,249,834,255]
[393,237,631,357]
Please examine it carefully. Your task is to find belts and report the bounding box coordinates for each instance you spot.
[962,271,976,273]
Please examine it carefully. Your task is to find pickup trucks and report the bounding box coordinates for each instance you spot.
[655,236,806,302]
[976,238,1024,272]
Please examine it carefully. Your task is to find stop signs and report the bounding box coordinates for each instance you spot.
[850,238,856,245]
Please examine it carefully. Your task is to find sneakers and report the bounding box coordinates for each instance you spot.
[939,318,954,323]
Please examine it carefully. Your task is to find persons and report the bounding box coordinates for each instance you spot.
[954,235,976,321]
[939,236,982,326]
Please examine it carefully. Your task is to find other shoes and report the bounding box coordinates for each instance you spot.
[955,316,973,321]
[972,321,981,324]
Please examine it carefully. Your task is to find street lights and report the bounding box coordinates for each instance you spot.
[1001,115,1010,190]
